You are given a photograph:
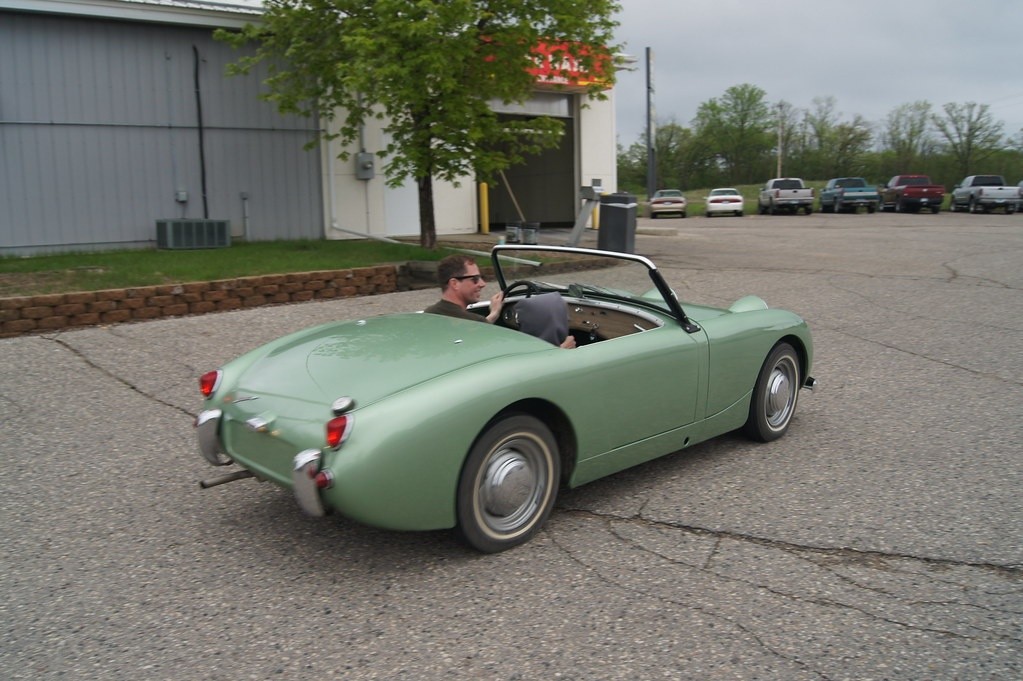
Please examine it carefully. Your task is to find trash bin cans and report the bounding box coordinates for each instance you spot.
[597,192,639,256]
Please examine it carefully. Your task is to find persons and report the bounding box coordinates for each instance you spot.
[513,291,569,347]
[422,253,576,351]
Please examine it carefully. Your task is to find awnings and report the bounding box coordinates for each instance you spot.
[480,30,615,94]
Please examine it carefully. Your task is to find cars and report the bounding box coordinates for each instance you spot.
[648,189,687,217]
[192,242,819,554]
[702,188,744,217]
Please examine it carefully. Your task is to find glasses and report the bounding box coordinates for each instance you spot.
[453,274,484,284]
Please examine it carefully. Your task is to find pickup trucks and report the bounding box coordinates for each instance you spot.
[820,178,880,214]
[879,175,945,214]
[758,178,815,214]
[949,175,1023,214]
